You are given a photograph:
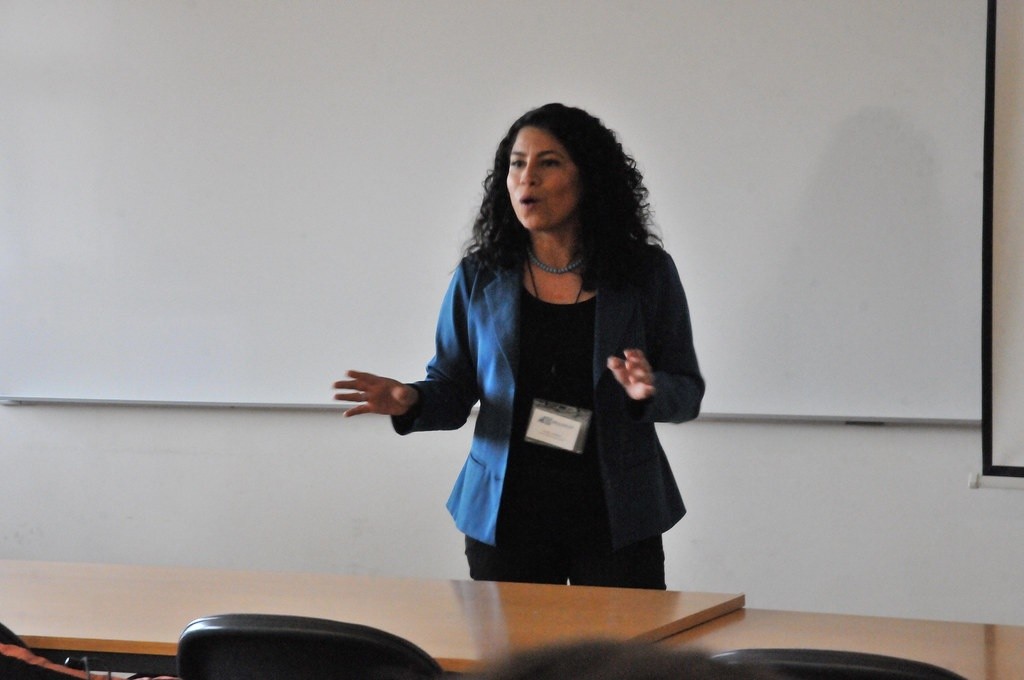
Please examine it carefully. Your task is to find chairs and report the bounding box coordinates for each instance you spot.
[175,612,447,680]
[708,649,968,680]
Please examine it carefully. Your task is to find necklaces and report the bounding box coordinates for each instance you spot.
[526,246,583,274]
[527,257,582,304]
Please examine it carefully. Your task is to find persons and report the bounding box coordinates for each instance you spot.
[332,101,705,591]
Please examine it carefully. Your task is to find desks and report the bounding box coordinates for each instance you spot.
[0,559,1024,680]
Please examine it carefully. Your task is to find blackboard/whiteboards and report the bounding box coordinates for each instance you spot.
[0,1,995,427]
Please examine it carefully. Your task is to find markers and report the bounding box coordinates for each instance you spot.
[846,420,886,427]
